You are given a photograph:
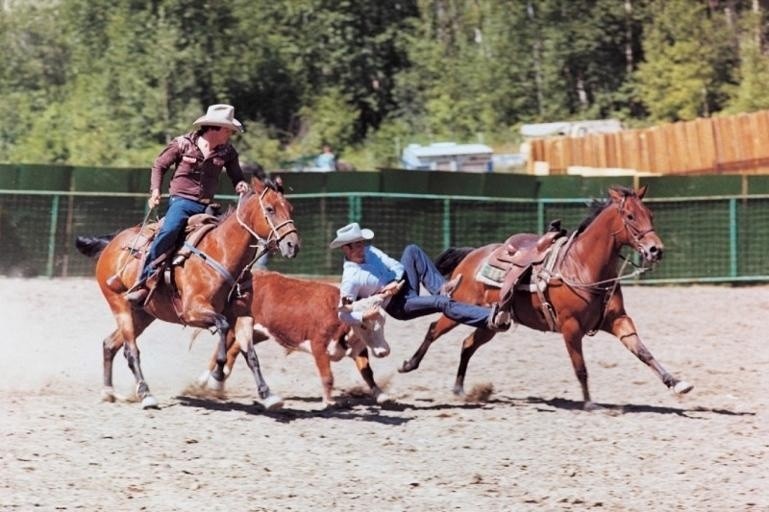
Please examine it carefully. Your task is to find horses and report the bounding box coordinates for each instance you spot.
[65,159,303,410]
[395,183,695,412]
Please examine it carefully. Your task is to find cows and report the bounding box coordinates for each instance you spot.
[197,265,409,408]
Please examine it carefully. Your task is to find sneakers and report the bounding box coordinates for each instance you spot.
[126,289,148,304]
[440,273,462,298]
[493,311,505,326]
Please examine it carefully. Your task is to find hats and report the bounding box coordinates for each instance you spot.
[193,104,242,131]
[329,222,374,249]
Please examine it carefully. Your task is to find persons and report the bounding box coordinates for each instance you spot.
[314,143,335,172]
[329,223,510,332]
[124,104,249,306]
